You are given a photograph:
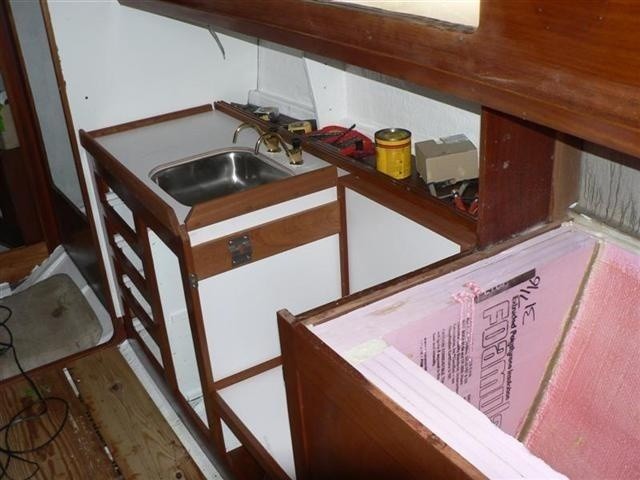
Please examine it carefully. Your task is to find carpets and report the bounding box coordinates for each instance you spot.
[0,273,102,382]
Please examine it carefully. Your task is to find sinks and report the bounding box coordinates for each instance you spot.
[147,146,296,207]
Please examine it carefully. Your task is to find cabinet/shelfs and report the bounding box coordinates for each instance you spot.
[87,151,207,446]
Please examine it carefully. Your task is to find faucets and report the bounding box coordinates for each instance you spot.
[254,132,303,165]
[232,122,282,152]
